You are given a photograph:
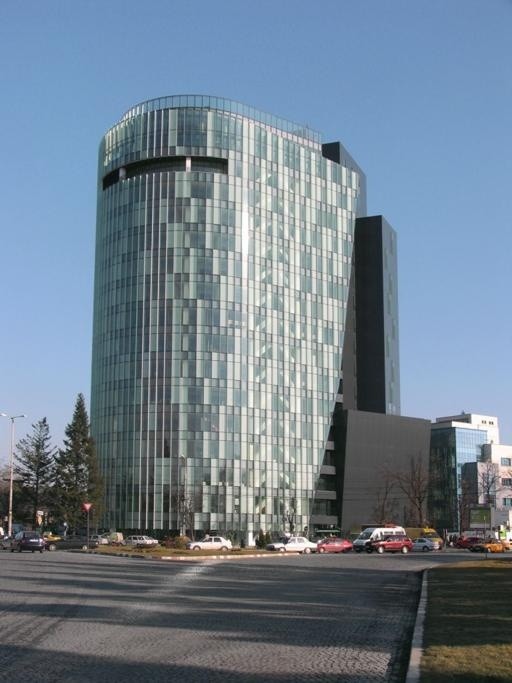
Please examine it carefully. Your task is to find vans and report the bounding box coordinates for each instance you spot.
[352,526,406,553]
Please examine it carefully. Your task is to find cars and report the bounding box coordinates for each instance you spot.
[318,536,354,554]
[453,534,512,554]
[186,534,234,551]
[266,535,318,554]
[0,530,161,550]
[410,536,440,551]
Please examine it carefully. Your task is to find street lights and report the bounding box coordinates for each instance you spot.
[180,452,188,535]
[0,412,27,538]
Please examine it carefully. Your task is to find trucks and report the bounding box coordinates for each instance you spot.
[405,525,444,550]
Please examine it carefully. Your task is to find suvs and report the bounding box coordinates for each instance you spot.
[10,530,45,554]
[364,533,413,553]
